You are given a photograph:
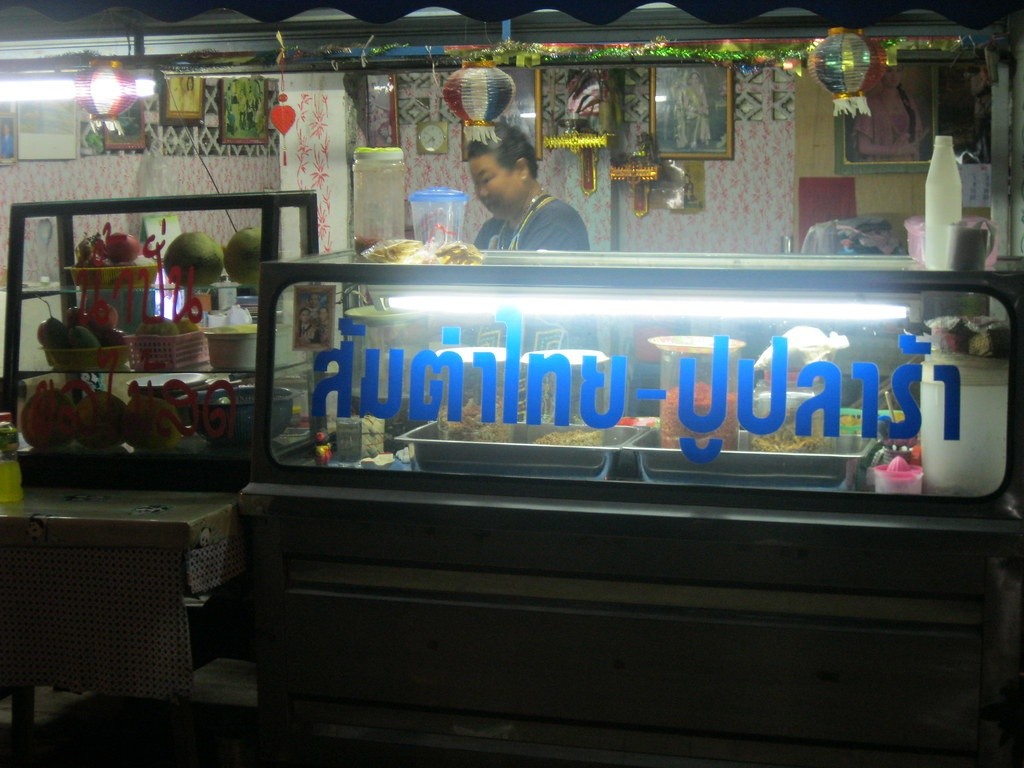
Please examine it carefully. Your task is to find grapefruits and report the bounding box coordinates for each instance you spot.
[18,388,183,451]
[162,231,224,288]
[222,226,267,284]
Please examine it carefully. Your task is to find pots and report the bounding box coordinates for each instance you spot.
[203,324,303,370]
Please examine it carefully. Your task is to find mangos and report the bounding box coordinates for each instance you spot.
[37,302,133,349]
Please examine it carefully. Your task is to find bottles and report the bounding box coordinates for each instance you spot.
[0,412,24,503]
[923,135,961,272]
[352,146,406,240]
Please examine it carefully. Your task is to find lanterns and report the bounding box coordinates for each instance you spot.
[442,61,517,145]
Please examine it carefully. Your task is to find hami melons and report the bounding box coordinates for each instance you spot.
[134,315,204,363]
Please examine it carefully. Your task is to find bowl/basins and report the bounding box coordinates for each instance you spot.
[904,214,1002,264]
[189,384,301,448]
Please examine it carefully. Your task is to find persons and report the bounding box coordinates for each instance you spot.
[183,76,198,112]
[225,87,264,136]
[297,294,322,344]
[467,125,592,414]
[852,65,922,162]
[670,72,711,150]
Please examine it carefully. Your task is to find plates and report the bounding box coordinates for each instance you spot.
[648,335,747,353]
[344,305,427,326]
[127,372,209,391]
[238,295,258,305]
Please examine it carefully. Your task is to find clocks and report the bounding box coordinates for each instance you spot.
[416,121,450,154]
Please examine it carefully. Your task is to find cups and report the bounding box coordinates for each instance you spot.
[882,437,923,465]
[335,417,362,463]
[196,293,211,312]
[944,223,987,272]
[873,456,925,494]
[408,185,469,243]
[781,235,792,254]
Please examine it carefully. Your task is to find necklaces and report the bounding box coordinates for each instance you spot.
[500,188,544,251]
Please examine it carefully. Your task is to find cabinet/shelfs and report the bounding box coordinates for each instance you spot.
[2,188,329,492]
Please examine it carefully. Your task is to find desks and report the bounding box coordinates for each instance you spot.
[0,487,245,768]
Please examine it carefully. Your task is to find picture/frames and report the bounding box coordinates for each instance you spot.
[218,79,270,145]
[158,78,207,127]
[368,75,400,148]
[649,66,734,160]
[103,100,146,150]
[833,63,940,174]
[0,111,18,165]
